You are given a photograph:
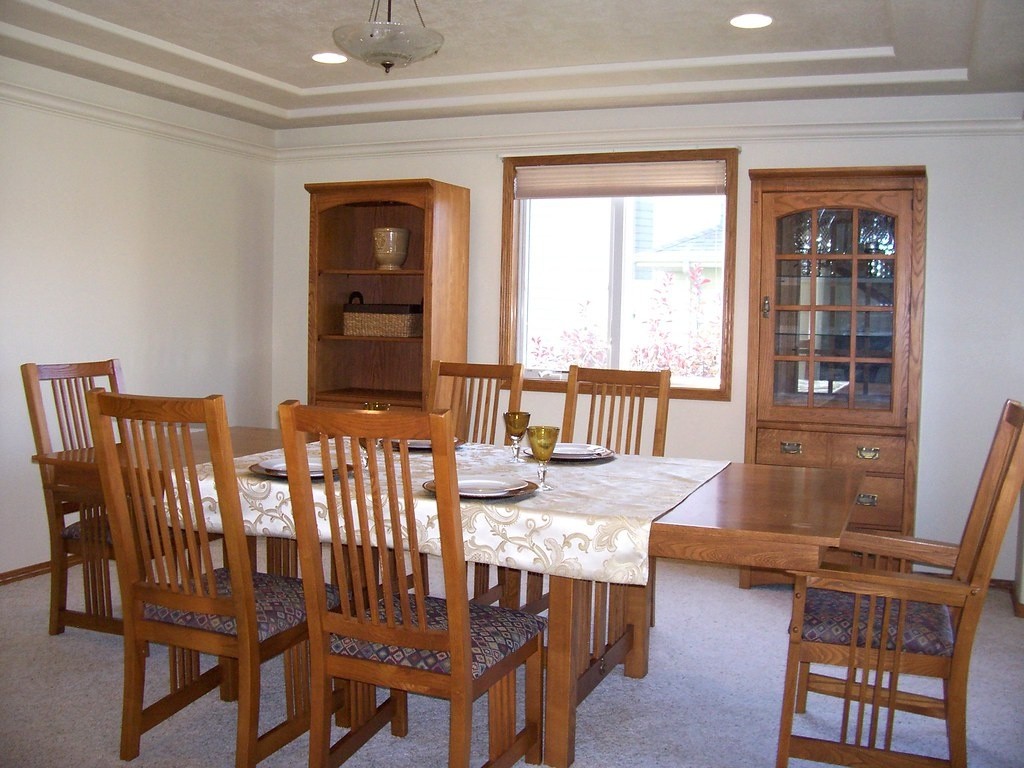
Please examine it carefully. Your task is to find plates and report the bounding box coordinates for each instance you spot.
[379,436,466,450]
[523,442,613,461]
[249,457,363,480]
[422,477,539,500]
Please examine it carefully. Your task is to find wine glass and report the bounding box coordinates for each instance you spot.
[527,426,561,492]
[503,412,531,463]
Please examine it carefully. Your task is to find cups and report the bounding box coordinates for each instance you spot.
[373,227,410,270]
[364,402,391,411]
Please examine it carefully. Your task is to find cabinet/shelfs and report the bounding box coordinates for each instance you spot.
[301,175,471,441]
[744,175,916,591]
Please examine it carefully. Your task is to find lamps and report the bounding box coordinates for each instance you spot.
[331,0,444,74]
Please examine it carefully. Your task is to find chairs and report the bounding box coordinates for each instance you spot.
[277,400,549,767]
[774,398,1023,767]
[387,359,525,598]
[19,357,223,656]
[499,365,672,674]
[85,386,351,768]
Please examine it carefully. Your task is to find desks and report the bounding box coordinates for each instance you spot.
[147,437,865,768]
